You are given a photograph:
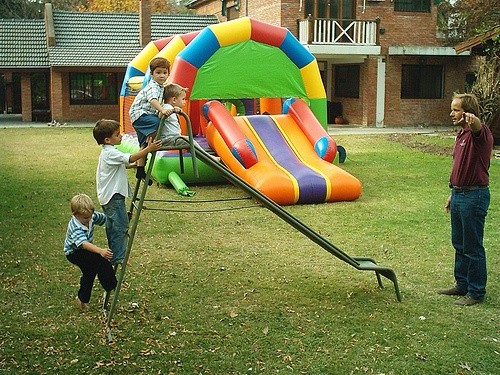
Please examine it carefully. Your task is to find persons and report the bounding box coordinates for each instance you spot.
[128,56,222,186]
[93,117,163,267]
[437,91,494,306]
[62,193,133,312]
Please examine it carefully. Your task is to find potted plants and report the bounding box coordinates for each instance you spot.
[335,115,343,124]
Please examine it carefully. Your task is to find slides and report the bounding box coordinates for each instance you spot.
[186,139,401,300]
[203,99,361,205]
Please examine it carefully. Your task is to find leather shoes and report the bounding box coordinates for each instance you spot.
[436,287,460,295]
[453,295,485,306]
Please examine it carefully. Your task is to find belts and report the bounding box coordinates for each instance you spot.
[450,185,488,191]
